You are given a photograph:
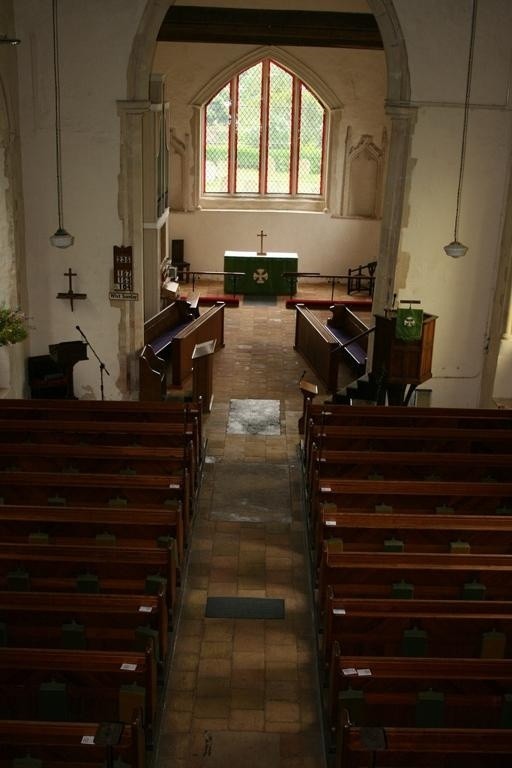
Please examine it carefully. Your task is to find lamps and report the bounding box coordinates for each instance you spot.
[443,0,479,258]
[47,0,74,250]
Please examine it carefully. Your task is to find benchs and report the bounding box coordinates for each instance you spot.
[2,396,210,768]
[298,401,512,768]
[293,304,370,392]
[144,301,225,391]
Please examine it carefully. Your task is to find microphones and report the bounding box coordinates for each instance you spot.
[75,325,87,338]
[400,300,421,304]
[391,293,396,308]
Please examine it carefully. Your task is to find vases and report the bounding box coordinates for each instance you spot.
[0,345,10,388]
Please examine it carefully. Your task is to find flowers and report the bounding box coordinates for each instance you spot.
[0,302,38,348]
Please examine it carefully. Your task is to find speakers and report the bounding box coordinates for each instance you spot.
[171,239,184,281]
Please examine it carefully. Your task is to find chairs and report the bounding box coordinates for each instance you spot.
[171,239,190,283]
